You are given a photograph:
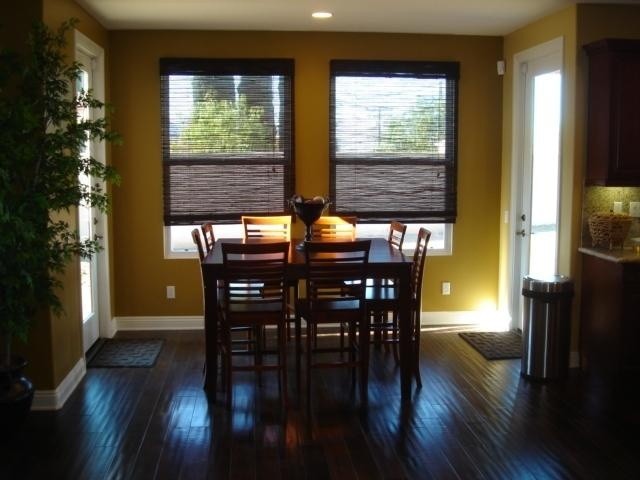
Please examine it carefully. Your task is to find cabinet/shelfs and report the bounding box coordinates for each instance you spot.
[579,256,639,412]
[586,36,638,190]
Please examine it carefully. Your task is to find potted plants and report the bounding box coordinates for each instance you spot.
[0,21,124,433]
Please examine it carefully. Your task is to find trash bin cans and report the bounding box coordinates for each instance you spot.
[519,274,575,382]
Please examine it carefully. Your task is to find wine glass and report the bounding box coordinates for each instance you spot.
[287,195,331,252]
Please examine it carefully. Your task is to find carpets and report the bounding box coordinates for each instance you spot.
[88,337,165,368]
[457,329,526,362]
